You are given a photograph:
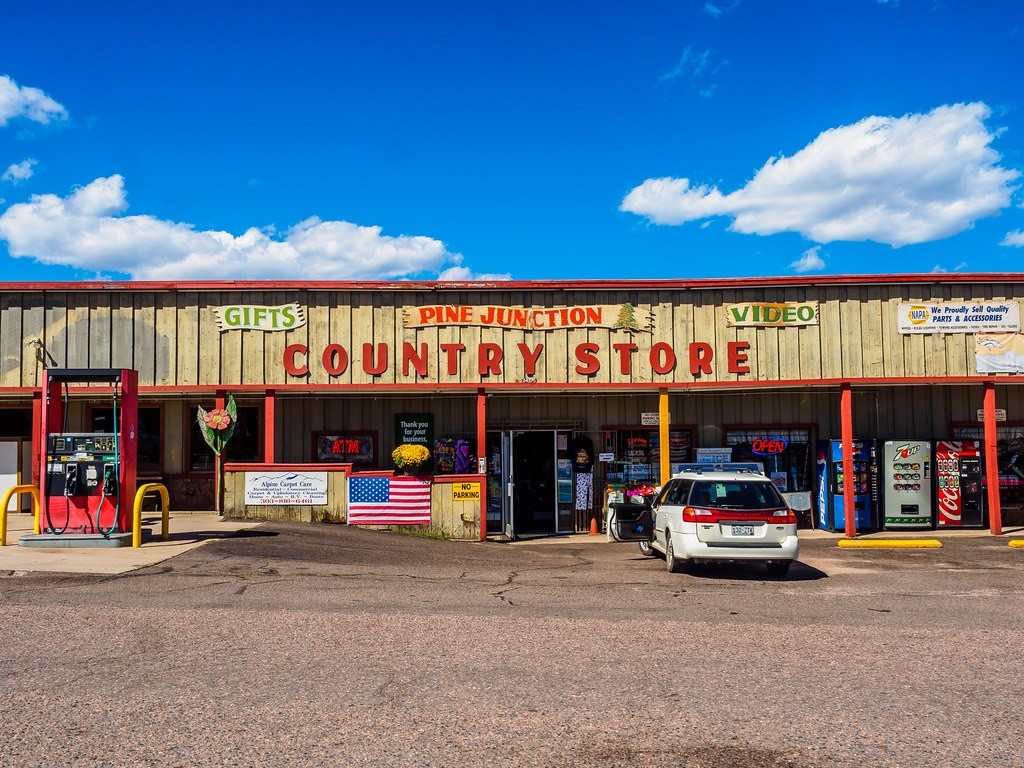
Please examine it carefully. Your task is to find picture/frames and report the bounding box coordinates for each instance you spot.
[311,430,379,469]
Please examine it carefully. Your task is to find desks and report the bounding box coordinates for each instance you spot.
[761,490,815,532]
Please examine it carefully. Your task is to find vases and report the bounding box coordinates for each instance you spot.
[403,464,422,476]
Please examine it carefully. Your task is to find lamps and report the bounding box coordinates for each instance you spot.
[23,338,43,387]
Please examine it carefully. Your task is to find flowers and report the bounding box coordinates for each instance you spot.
[391,443,431,469]
[196,394,238,515]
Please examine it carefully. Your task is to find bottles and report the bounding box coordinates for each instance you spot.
[938,460,959,488]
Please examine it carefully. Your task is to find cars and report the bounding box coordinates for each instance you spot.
[606,469,799,577]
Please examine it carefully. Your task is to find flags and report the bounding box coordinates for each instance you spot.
[347,474,432,526]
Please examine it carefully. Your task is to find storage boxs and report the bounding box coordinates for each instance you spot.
[982,475,1019,487]
[692,447,732,463]
[558,479,573,503]
[557,459,572,480]
